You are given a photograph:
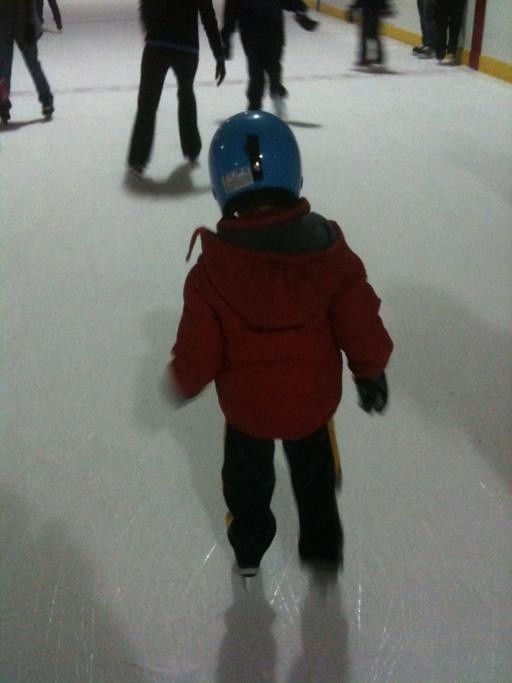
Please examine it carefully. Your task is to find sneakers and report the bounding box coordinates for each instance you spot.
[42,103,54,115]
[235,546,261,577]
[413,45,432,56]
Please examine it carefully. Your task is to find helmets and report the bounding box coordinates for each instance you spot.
[209,111,302,209]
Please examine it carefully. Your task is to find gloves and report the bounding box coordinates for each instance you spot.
[359,370,387,413]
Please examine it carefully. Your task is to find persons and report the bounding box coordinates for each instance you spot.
[161,109,395,577]
